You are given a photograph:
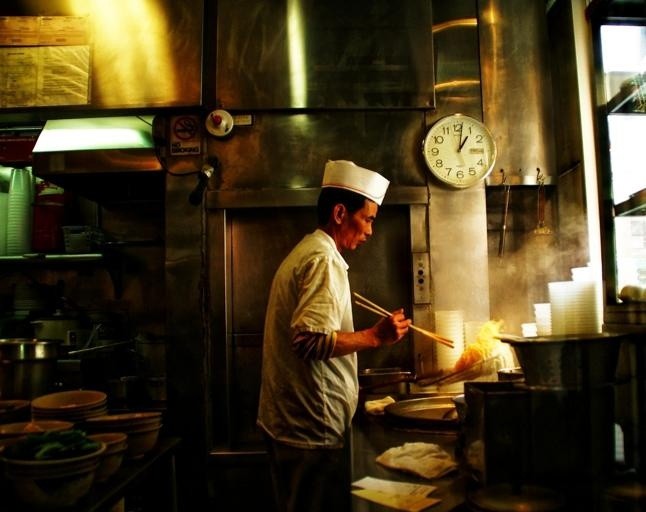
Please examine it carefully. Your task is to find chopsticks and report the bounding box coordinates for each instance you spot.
[353,293,453,349]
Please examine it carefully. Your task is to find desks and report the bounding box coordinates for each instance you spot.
[71,434,184,509]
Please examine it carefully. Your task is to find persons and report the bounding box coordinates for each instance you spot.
[258,158,412,512]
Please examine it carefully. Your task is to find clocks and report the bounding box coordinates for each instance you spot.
[420,113,498,192]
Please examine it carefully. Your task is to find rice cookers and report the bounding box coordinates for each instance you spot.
[29,306,80,345]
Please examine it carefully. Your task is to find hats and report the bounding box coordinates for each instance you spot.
[321,159,390,206]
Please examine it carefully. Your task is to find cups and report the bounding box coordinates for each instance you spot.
[433,267,602,371]
[5,167,35,254]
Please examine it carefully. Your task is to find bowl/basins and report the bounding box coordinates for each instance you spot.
[500,328,623,387]
[380,391,460,458]
[1,338,162,510]
[620,285,646,302]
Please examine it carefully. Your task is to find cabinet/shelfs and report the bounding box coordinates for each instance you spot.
[584,4,646,332]
